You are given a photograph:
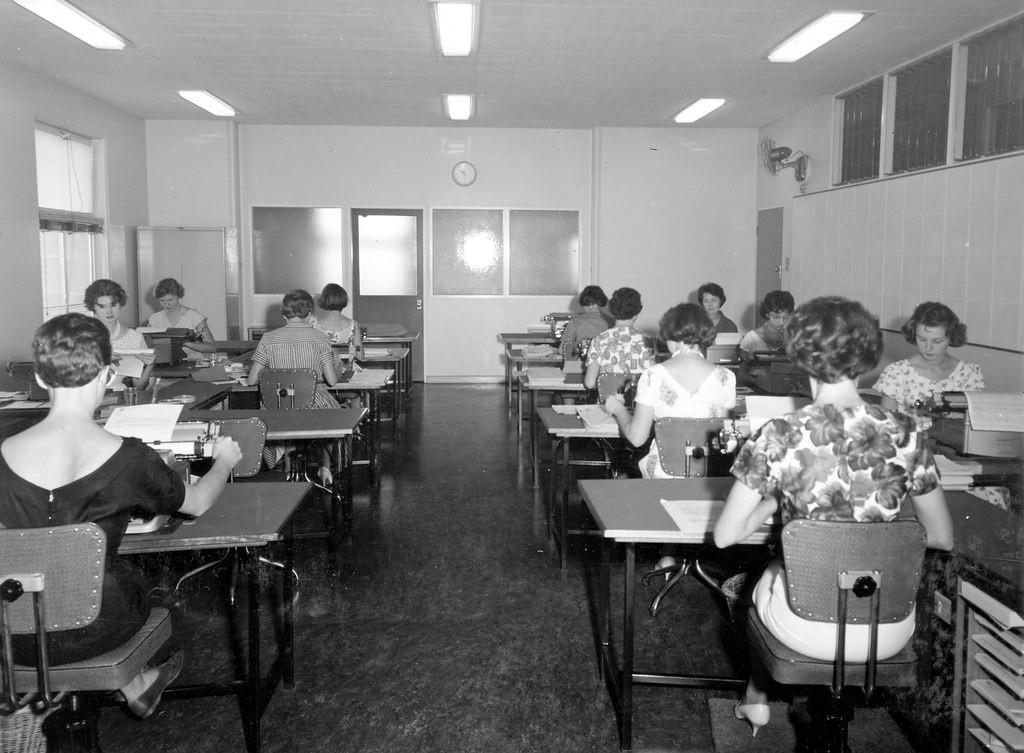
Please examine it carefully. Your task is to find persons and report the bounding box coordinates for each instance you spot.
[712,296,954,737]
[310,283,365,439]
[604,303,737,581]
[697,283,737,333]
[738,289,797,362]
[145,278,217,367]
[247,289,343,487]
[0,312,244,716]
[84,279,157,392]
[557,286,614,354]
[871,302,1008,511]
[583,287,661,405]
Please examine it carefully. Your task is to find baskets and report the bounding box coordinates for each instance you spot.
[0,703,61,753]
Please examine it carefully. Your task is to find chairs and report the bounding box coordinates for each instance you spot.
[1,522,173,752]
[167,416,300,610]
[594,371,642,478]
[561,340,596,404]
[255,366,344,536]
[644,411,744,622]
[746,518,928,752]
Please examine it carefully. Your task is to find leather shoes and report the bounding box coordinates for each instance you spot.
[128,652,183,718]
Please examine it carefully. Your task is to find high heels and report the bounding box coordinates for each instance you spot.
[735,700,770,737]
[656,556,678,581]
[317,466,332,488]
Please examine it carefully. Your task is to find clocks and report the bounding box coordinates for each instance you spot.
[452,161,476,185]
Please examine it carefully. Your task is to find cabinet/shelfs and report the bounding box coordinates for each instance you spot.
[133,227,225,341]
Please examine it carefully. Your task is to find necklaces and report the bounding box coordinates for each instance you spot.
[109,318,121,341]
[671,348,705,358]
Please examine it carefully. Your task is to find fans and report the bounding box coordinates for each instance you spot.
[759,137,808,180]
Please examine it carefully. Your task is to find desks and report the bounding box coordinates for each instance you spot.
[1,332,1023,752]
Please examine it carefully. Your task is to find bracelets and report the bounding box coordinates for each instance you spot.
[763,321,784,348]
